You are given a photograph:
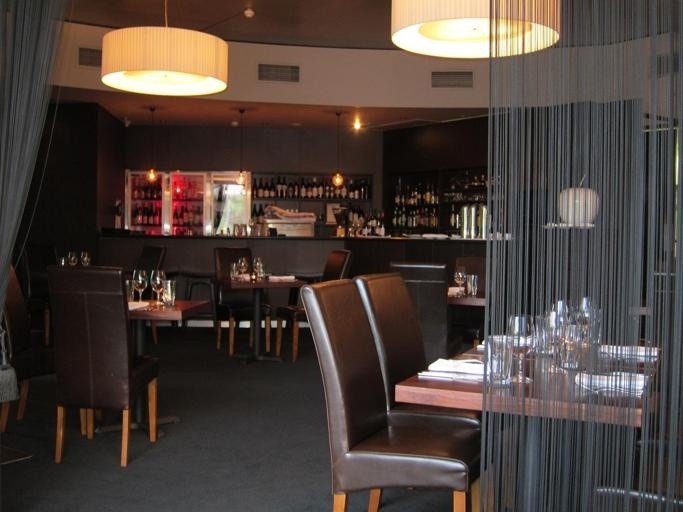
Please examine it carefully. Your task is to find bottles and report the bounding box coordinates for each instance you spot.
[336,224,345,237]
[251,203,264,223]
[250,271,256,282]
[249,176,370,200]
[226,227,230,237]
[345,200,388,238]
[131,178,203,236]
[472,329,481,347]
[391,174,488,230]
[215,185,224,227]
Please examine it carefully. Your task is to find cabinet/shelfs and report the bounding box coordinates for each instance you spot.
[252,174,373,238]
[384,115,500,234]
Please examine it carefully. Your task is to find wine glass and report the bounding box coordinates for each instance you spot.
[236,257,249,274]
[150,268,166,307]
[453,271,465,288]
[132,268,148,301]
[248,218,255,238]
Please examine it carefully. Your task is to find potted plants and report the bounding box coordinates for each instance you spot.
[558,174,600,224]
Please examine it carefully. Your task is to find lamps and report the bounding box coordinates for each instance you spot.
[332,112,344,186]
[101,0,228,97]
[147,107,156,181]
[237,109,245,185]
[390,0,562,59]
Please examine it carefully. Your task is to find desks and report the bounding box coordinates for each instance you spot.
[395,338,661,511]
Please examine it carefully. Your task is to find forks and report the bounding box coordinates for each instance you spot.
[503,295,598,386]
[476,344,536,358]
[576,381,642,397]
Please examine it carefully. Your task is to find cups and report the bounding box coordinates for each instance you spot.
[124,280,136,302]
[465,273,476,296]
[162,279,177,306]
[57,250,90,266]
[251,255,267,278]
[233,224,247,238]
[484,335,513,388]
[230,262,238,280]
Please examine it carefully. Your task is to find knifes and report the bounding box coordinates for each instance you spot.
[417,372,482,384]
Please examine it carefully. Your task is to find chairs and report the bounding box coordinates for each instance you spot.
[289,269,519,511]
[2,228,350,470]
